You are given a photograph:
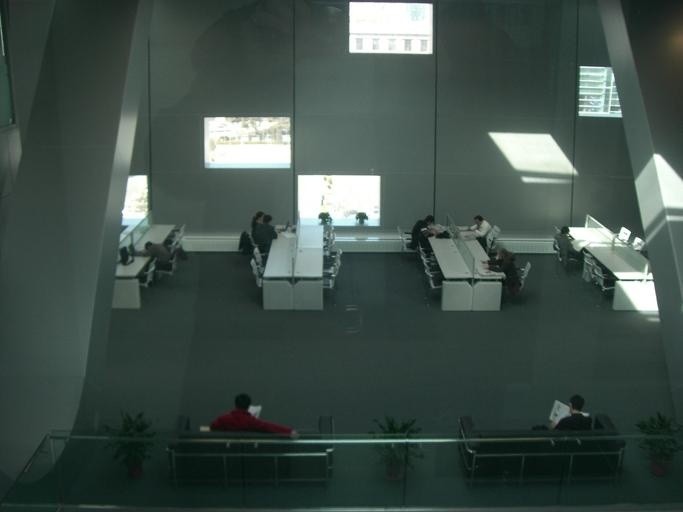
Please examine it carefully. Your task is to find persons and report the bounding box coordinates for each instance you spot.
[138,241,172,289]
[558,224,585,267]
[479,246,522,298]
[208,390,299,438]
[406,214,437,251]
[466,214,494,250]
[552,394,592,433]
[252,216,279,261]
[250,211,265,241]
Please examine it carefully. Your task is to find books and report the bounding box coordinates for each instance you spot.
[572,236,591,254]
[247,406,262,416]
[549,399,589,424]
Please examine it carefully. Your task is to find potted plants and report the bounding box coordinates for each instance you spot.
[362,416,424,479]
[318,212,332,225]
[101,411,163,478]
[633,410,682,475]
[356,212,369,224]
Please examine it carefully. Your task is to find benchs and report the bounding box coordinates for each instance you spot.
[458,413,623,490]
[167,415,334,487]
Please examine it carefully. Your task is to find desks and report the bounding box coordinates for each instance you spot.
[262,224,324,311]
[569,226,660,310]
[110,221,177,310]
[427,225,506,312]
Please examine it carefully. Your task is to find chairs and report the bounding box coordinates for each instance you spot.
[396,227,448,309]
[490,246,532,295]
[323,221,343,311]
[241,230,264,311]
[138,223,185,287]
[552,226,582,274]
